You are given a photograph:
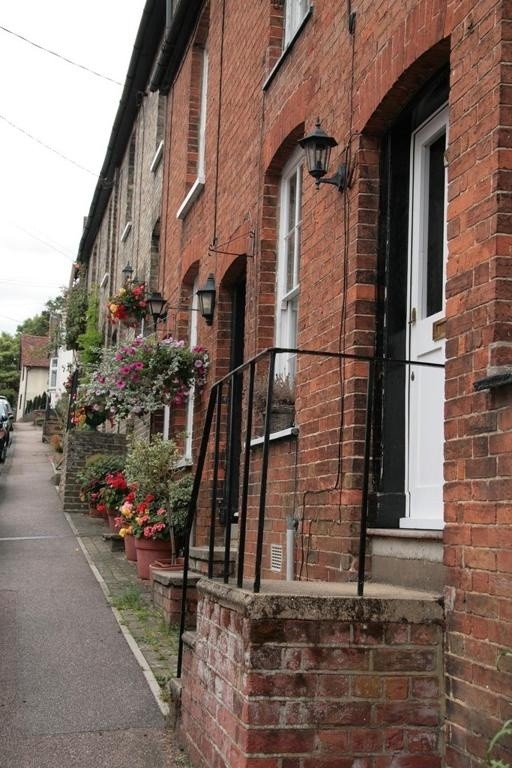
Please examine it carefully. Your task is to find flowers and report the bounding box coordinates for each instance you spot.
[75,284,210,539]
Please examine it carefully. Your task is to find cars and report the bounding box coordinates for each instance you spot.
[0,394,16,462]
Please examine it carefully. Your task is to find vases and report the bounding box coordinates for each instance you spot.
[118,534,170,580]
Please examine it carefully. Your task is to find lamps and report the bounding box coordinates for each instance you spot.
[296,116,348,195]
[193,271,215,324]
[122,260,134,283]
